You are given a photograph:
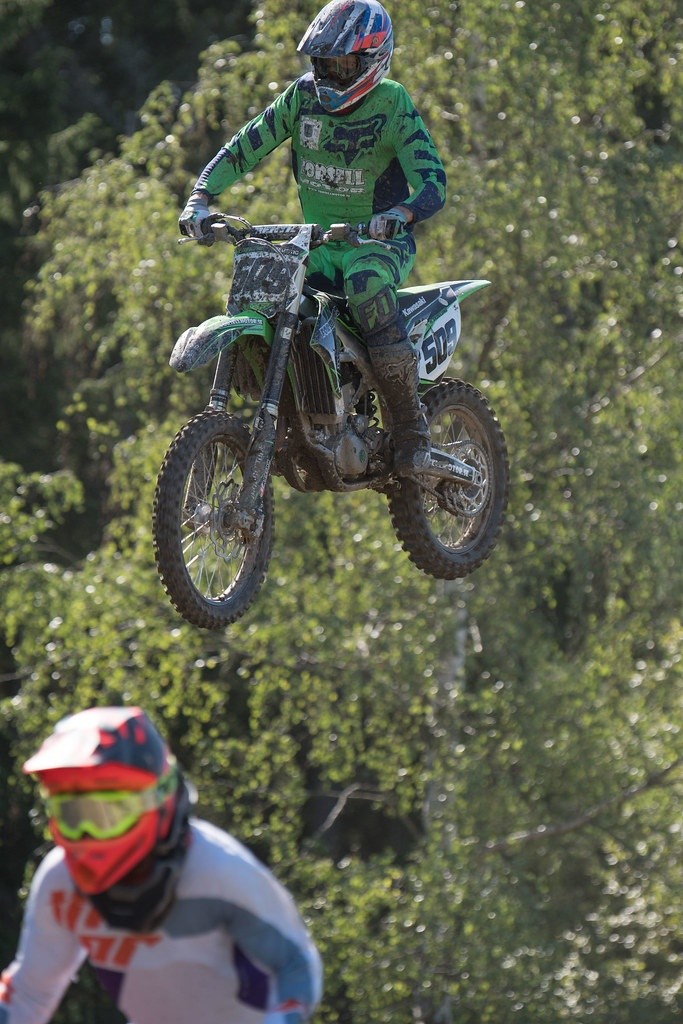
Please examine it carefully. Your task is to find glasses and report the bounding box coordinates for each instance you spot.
[315,55,366,81]
[45,756,179,838]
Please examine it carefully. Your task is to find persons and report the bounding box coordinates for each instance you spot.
[0,706,322,1024]
[179,0,446,477]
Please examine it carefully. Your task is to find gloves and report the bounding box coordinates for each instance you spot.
[369,209,407,241]
[178,198,211,239]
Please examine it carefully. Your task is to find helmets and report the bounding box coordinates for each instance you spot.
[22,705,179,892]
[296,0,393,113]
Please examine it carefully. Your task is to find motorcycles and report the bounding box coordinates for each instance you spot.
[151,213,510,631]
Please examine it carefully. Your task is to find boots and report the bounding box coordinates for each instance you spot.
[368,339,431,478]
